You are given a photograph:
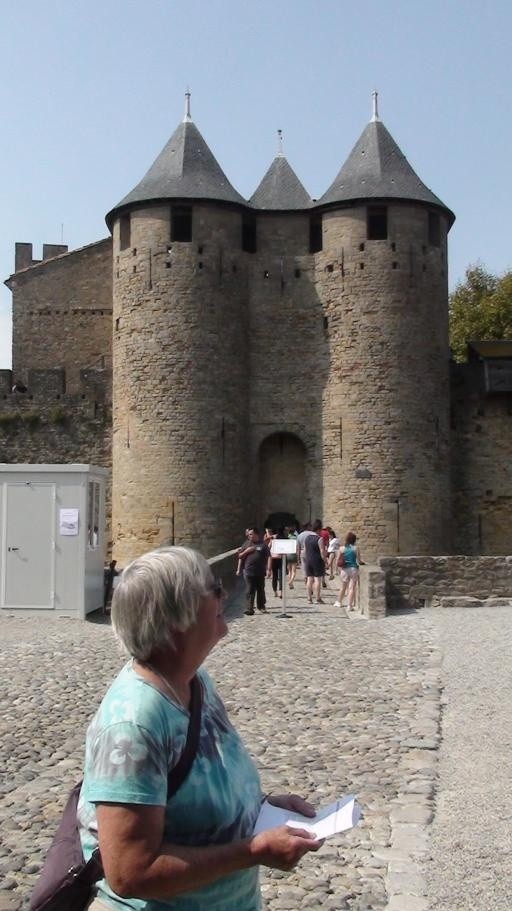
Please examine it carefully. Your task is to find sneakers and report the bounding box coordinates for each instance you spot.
[243,605,267,616]
[273,576,324,605]
[332,602,353,612]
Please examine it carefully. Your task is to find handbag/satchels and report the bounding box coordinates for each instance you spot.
[26,777,98,911]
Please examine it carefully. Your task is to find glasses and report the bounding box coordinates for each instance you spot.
[205,576,225,598]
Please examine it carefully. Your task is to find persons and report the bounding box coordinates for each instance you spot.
[236,516,366,617]
[77,544,325,911]
